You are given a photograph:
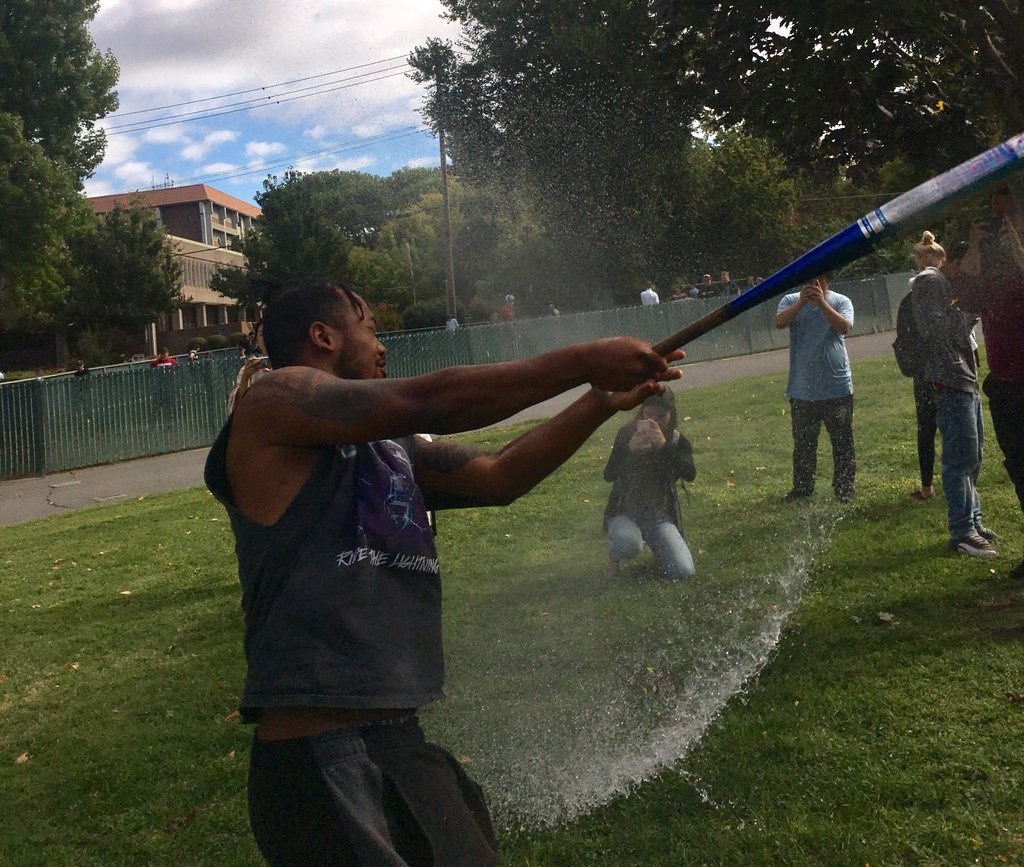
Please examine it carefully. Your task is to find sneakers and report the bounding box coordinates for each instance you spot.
[907,488,941,502]
[783,488,814,503]
[834,488,856,502]
[1010,560,1024,578]
[949,533,1000,561]
[976,525,999,540]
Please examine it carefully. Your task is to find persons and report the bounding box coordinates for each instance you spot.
[743,276,756,294]
[698,274,721,298]
[75,360,88,376]
[152,348,178,369]
[689,283,700,298]
[966,182,1024,580]
[226,316,275,416]
[913,239,1004,557]
[444,313,459,331]
[757,276,763,283]
[187,346,200,365]
[601,385,695,581]
[911,231,981,501]
[501,295,515,322]
[720,271,741,298]
[201,280,688,867]
[638,280,660,306]
[240,331,256,365]
[774,266,856,504]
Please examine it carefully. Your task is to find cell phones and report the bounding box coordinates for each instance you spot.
[975,218,1005,233]
[637,420,653,448]
[806,278,816,286]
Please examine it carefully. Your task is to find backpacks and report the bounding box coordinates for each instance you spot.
[890,291,935,377]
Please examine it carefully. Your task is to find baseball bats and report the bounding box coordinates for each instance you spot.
[649,132,1024,360]
